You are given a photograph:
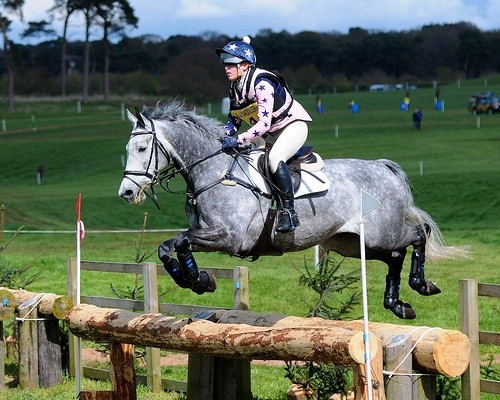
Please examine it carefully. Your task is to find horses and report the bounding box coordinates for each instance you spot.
[117,95,478,320]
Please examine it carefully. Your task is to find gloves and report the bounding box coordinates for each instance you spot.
[222,137,239,154]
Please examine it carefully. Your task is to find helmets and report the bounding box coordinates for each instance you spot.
[216,36,256,83]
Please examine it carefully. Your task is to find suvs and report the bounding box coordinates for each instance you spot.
[469,92,500,116]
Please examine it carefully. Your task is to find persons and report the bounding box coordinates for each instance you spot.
[316,96,323,113]
[403,92,410,110]
[215,41,308,233]
[433,84,440,107]
[413,109,423,129]
[349,97,355,112]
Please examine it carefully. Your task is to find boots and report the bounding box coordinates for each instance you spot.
[268,161,300,232]
